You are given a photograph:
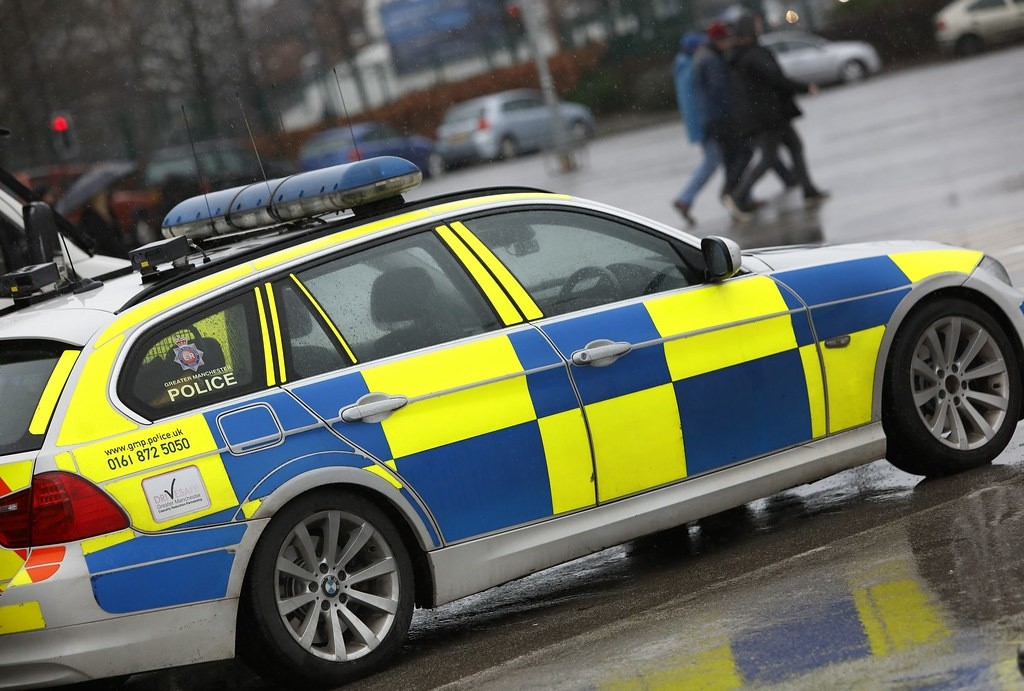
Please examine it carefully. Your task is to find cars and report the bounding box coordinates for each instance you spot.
[933,0,1024,57]
[296,118,438,180]
[432,85,598,166]
[758,29,885,88]
[0,150,1023,691]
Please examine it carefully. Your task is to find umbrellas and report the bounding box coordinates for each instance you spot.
[53,160,138,215]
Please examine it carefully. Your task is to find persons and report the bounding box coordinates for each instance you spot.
[39,184,124,251]
[672,11,830,228]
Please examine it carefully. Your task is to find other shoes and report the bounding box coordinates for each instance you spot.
[807,191,827,202]
[785,177,799,185]
[745,202,765,211]
[673,203,695,226]
[725,196,753,222]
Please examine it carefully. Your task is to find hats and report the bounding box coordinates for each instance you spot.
[704,24,730,42]
